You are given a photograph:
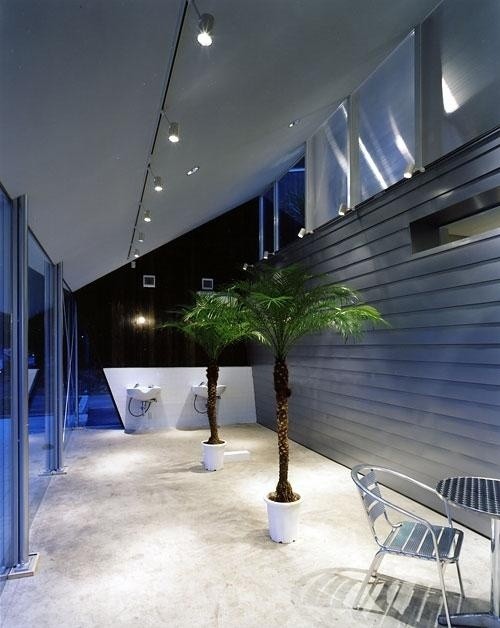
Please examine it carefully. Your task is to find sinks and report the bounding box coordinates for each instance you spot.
[126,386,161,402]
[191,385,227,399]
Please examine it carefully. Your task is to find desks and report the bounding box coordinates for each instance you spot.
[436,475,500,628]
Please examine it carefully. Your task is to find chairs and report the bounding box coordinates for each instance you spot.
[350,462,466,627]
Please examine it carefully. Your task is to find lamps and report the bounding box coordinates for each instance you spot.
[126,0,215,269]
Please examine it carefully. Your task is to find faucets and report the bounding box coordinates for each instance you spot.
[148,384,154,388]
